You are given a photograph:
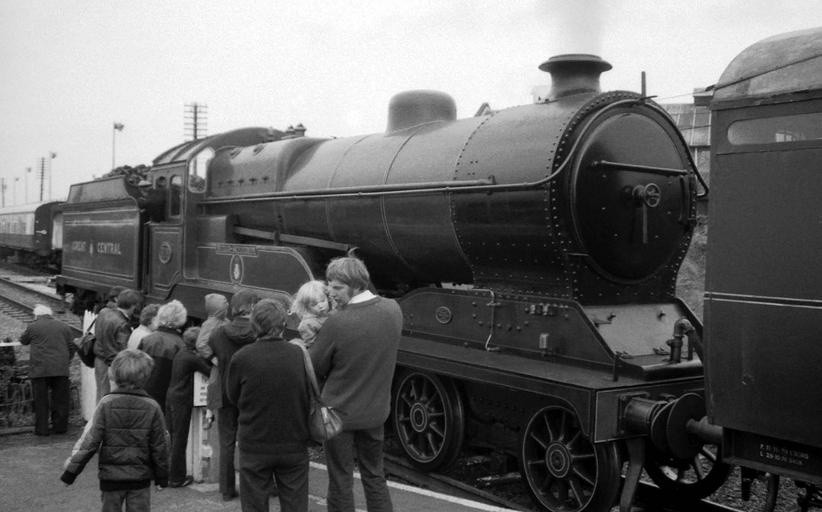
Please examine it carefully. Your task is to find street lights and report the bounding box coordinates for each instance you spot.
[183,101,209,141]
[12,175,20,210]
[111,121,125,173]
[48,151,59,200]
[1,176,7,206]
[24,166,34,205]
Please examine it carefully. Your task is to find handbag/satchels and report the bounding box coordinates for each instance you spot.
[307,402,345,443]
[72,316,95,368]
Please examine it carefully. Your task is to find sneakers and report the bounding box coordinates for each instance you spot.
[177,475,192,487]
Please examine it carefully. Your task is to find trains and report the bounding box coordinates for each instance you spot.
[1,199,63,272]
[47,25,822,507]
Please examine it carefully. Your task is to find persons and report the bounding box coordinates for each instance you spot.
[19,305,75,437]
[290,280,343,348]
[62,349,172,512]
[226,298,311,512]
[307,247,403,512]
[90,289,259,503]
